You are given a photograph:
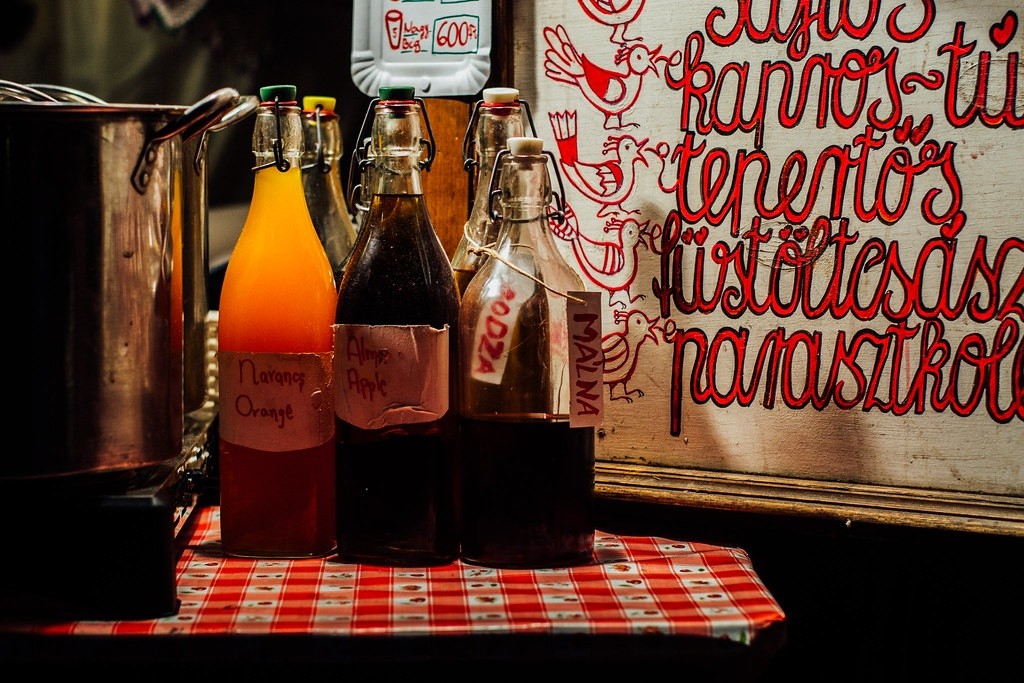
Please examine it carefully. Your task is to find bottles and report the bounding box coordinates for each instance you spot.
[451,87,595,570]
[332,86,461,568]
[218,85,358,559]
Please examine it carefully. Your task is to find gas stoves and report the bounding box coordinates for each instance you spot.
[3,408,213,627]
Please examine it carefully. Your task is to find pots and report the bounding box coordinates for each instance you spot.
[0,79,257,494]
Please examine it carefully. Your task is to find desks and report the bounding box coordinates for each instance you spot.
[0,504,786,645]
